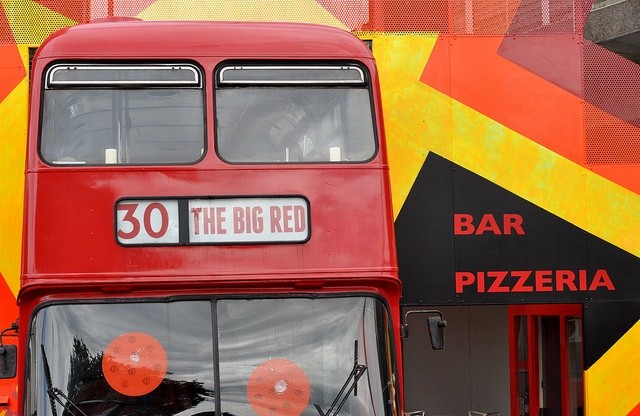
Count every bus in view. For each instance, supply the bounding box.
[17,14,447,415]
